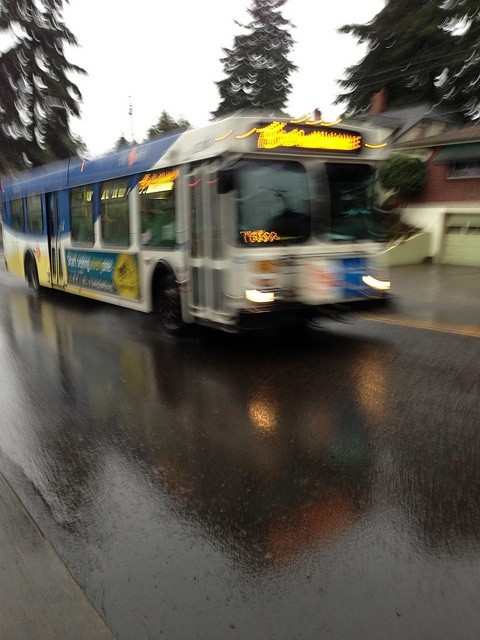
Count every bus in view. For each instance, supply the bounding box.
[1,114,396,335]
